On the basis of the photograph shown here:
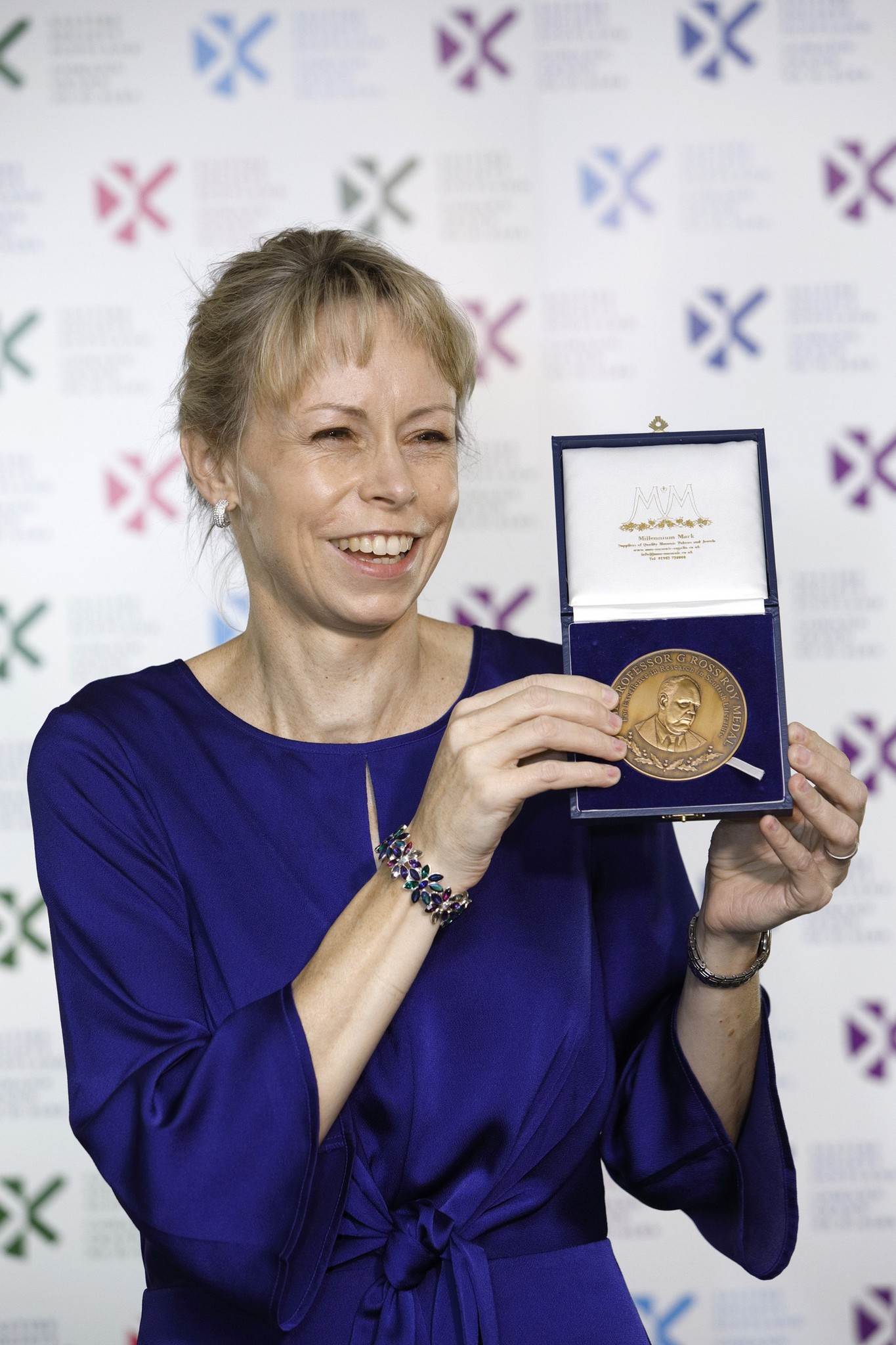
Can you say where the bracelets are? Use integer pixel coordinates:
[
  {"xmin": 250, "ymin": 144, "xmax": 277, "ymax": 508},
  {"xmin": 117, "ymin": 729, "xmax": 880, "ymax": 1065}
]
[
  {"xmin": 374, "ymin": 825, "xmax": 472, "ymax": 928},
  {"xmin": 688, "ymin": 912, "xmax": 771, "ymax": 988}
]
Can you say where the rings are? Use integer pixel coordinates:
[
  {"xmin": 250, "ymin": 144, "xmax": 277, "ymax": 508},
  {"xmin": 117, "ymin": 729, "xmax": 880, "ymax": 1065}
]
[{"xmin": 825, "ymin": 841, "xmax": 859, "ymax": 861}]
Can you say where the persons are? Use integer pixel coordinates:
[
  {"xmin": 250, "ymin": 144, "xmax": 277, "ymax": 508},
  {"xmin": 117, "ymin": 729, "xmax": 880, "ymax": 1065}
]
[{"xmin": 26, "ymin": 227, "xmax": 869, "ymax": 1345}]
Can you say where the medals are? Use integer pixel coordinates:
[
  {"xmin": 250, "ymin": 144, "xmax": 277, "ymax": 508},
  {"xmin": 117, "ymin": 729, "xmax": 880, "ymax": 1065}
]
[{"xmin": 609, "ymin": 649, "xmax": 747, "ymax": 780}]
